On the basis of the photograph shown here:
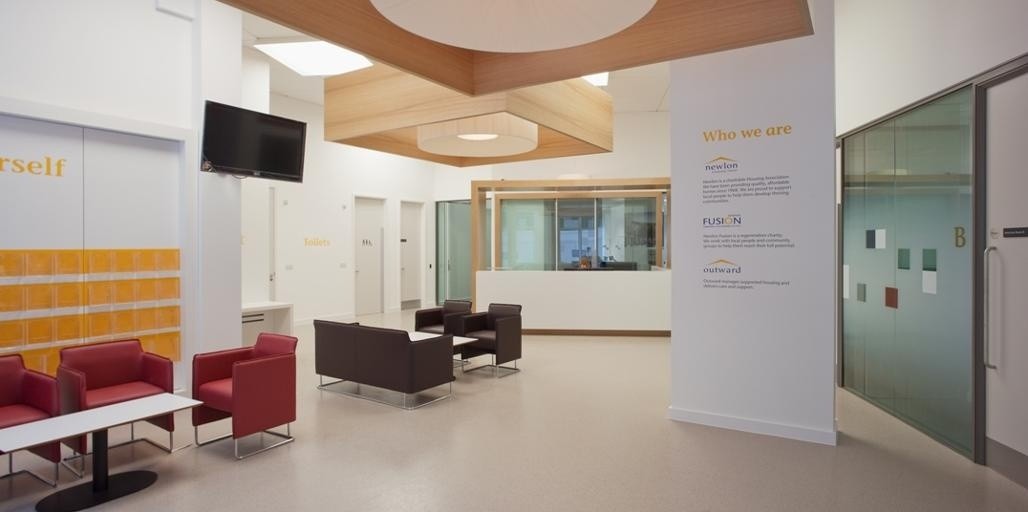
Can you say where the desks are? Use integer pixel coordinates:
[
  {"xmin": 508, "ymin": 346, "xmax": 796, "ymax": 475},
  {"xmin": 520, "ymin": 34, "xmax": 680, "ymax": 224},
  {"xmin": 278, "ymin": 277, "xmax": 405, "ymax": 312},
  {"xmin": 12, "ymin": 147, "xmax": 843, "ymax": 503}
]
[
  {"xmin": 0, "ymin": 391, "xmax": 205, "ymax": 512},
  {"xmin": 407, "ymin": 331, "xmax": 480, "ymax": 347}
]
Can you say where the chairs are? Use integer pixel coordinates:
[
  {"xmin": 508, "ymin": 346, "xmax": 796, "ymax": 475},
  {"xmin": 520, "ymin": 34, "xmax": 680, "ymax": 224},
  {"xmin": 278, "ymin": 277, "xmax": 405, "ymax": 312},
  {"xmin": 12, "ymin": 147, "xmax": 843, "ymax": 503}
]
[
  {"xmin": 192, "ymin": 331, "xmax": 299, "ymax": 460},
  {"xmin": 56, "ymin": 338, "xmax": 175, "ymax": 479},
  {"xmin": 414, "ymin": 299, "xmax": 473, "ymax": 369},
  {"xmin": 0, "ymin": 354, "xmax": 63, "ymax": 489},
  {"xmin": 460, "ymin": 303, "xmax": 522, "ymax": 378}
]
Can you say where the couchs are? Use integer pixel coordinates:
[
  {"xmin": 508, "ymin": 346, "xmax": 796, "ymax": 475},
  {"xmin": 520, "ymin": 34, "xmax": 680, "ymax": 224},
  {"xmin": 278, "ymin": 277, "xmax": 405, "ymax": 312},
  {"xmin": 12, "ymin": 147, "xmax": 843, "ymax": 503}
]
[{"xmin": 313, "ymin": 318, "xmax": 456, "ymax": 411}]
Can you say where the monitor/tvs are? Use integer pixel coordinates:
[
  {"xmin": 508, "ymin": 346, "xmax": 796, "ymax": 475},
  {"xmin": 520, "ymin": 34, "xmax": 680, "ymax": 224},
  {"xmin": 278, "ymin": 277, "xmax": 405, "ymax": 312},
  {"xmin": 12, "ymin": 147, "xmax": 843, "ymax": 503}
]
[{"xmin": 200, "ymin": 100, "xmax": 306, "ymax": 183}]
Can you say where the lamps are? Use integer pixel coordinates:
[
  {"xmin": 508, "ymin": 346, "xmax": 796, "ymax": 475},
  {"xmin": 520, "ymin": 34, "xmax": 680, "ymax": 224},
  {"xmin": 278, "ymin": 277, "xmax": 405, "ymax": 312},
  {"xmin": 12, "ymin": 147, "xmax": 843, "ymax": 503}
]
[
  {"xmin": 416, "ymin": 112, "xmax": 539, "ymax": 158},
  {"xmin": 251, "ymin": 34, "xmax": 375, "ymax": 78}
]
